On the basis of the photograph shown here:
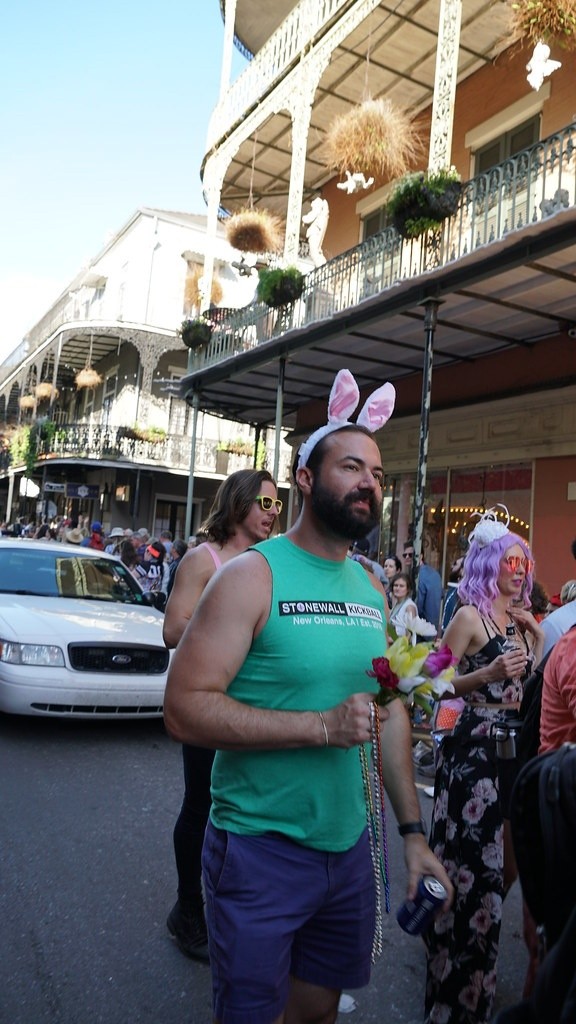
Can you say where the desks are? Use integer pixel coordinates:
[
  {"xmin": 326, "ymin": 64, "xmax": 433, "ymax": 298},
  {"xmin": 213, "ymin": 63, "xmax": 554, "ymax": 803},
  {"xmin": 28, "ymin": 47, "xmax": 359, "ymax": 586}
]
[{"xmin": 203, "ymin": 308, "xmax": 256, "ymax": 349}]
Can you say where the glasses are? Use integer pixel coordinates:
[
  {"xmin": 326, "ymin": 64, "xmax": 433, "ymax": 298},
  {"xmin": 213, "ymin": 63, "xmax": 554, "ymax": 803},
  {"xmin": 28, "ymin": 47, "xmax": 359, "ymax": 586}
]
[
  {"xmin": 501, "ymin": 556, "xmax": 534, "ymax": 576},
  {"xmin": 403, "ymin": 553, "xmax": 413, "ymax": 558},
  {"xmin": 255, "ymin": 496, "xmax": 283, "ymax": 515}
]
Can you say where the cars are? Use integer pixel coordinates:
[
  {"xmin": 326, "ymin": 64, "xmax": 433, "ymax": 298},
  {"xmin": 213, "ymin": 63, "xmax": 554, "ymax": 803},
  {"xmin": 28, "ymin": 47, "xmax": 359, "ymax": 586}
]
[{"xmin": 0, "ymin": 539, "xmax": 177, "ymax": 718}]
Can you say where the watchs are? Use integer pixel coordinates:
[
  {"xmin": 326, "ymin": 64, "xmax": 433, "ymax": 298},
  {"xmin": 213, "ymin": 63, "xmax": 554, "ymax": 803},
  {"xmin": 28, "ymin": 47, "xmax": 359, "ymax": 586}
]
[{"xmin": 399, "ymin": 818, "xmax": 426, "ymax": 836}]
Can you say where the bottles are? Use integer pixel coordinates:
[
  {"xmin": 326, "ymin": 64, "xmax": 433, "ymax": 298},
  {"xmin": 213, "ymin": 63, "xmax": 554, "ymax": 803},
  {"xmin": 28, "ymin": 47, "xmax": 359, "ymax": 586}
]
[
  {"xmin": 501, "ymin": 623, "xmax": 528, "ymax": 676},
  {"xmin": 490, "ymin": 715, "xmax": 526, "ymax": 758}
]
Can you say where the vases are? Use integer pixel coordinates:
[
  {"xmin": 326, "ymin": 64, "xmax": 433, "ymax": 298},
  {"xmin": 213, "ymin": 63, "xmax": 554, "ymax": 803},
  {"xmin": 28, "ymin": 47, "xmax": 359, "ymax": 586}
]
[
  {"xmin": 183, "ymin": 325, "xmax": 211, "ymax": 348},
  {"xmin": 392, "ymin": 181, "xmax": 459, "ymax": 240}
]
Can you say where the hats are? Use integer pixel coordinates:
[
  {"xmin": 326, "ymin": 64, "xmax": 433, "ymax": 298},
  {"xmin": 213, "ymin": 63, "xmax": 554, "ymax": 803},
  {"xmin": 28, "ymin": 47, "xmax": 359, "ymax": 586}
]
[
  {"xmin": 66, "ymin": 528, "xmax": 83, "ymax": 543},
  {"xmin": 92, "ymin": 522, "xmax": 101, "ymax": 529},
  {"xmin": 352, "ymin": 538, "xmax": 370, "ymax": 553},
  {"xmin": 108, "ymin": 528, "xmax": 124, "ymax": 537}
]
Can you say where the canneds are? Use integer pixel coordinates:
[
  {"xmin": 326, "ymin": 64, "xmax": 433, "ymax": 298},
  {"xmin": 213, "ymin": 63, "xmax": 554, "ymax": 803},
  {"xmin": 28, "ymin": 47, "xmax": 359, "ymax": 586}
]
[{"xmin": 395, "ymin": 876, "xmax": 448, "ymax": 936}]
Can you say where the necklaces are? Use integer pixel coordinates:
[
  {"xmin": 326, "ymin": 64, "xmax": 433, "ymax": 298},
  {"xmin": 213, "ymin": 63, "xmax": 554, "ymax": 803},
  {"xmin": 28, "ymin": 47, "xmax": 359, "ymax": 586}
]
[
  {"xmin": 390, "ymin": 599, "xmax": 406, "ymax": 619},
  {"xmin": 488, "ymin": 612, "xmax": 513, "ymax": 635},
  {"xmin": 358, "ymin": 701, "xmax": 390, "ymax": 963}
]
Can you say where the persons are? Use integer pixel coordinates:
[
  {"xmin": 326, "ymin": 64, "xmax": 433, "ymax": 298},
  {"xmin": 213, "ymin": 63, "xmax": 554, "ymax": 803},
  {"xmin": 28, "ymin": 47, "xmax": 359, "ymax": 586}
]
[
  {"xmin": 423, "ymin": 520, "xmax": 545, "ymax": 1024},
  {"xmin": 0, "ymin": 516, "xmax": 208, "ymax": 603},
  {"xmin": 0, "ymin": 434, "xmax": 10, "ymax": 451},
  {"xmin": 250, "ymin": 258, "xmax": 273, "ymax": 344},
  {"xmin": 538, "ymin": 538, "xmax": 576, "ymax": 659},
  {"xmin": 164, "ymin": 429, "xmax": 453, "ymax": 1024},
  {"xmin": 529, "ymin": 580, "xmax": 576, "ymax": 622},
  {"xmin": 163, "ymin": 469, "xmax": 281, "ymax": 964},
  {"xmin": 350, "ymin": 538, "xmax": 464, "ymax": 777},
  {"xmin": 519, "ymin": 625, "xmax": 576, "ymax": 999},
  {"xmin": 302, "ymin": 198, "xmax": 330, "ymax": 283}
]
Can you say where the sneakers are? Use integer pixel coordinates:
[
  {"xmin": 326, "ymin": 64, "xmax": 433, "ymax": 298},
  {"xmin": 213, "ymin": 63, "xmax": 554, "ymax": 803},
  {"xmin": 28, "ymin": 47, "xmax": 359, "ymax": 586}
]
[{"xmin": 166, "ymin": 901, "xmax": 210, "ymax": 963}]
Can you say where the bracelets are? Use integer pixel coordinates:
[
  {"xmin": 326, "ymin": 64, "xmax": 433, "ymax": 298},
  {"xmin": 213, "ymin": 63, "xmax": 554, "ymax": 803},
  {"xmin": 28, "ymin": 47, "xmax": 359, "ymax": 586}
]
[{"xmin": 318, "ymin": 711, "xmax": 328, "ymax": 747}]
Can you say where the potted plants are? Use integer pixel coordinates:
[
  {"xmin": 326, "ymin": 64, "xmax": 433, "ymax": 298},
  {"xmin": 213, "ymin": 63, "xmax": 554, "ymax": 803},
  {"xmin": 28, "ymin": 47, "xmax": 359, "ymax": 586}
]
[
  {"xmin": 214, "ymin": 439, "xmax": 254, "ymax": 475},
  {"xmin": 257, "ymin": 266, "xmax": 304, "ymax": 308}
]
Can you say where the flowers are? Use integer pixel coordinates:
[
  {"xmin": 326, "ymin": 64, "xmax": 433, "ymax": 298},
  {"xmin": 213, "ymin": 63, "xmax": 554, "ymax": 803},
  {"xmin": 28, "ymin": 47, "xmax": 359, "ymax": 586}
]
[
  {"xmin": 385, "ymin": 164, "xmax": 462, "ymax": 257},
  {"xmin": 368, "ymin": 610, "xmax": 459, "ymax": 718},
  {"xmin": 180, "ymin": 319, "xmax": 209, "ymax": 338}
]
